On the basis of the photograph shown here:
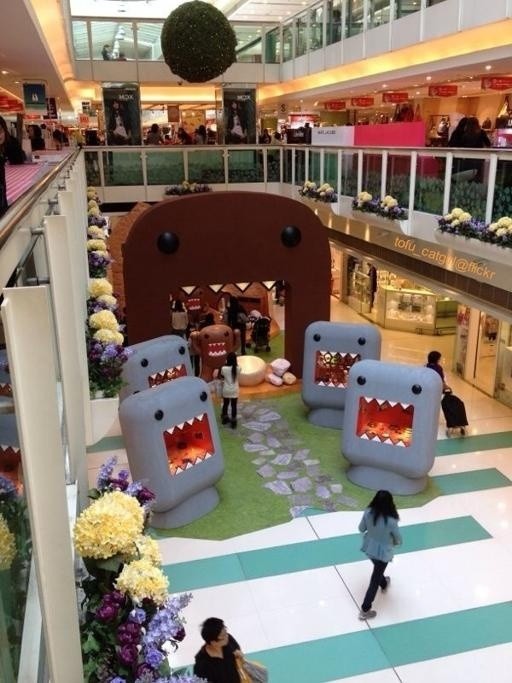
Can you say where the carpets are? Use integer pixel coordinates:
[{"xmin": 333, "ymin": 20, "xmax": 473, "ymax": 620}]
[{"xmin": 144, "ymin": 389, "xmax": 445, "ymax": 542}]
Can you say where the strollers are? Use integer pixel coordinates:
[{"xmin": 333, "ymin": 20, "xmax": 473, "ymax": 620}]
[{"xmin": 246, "ymin": 314, "xmax": 273, "ymax": 353}]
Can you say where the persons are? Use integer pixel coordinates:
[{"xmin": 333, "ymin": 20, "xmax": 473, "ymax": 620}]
[
  {"xmin": 226, "ymin": 296, "xmax": 272, "ymax": 355},
  {"xmin": 423, "ymin": 350, "xmax": 445, "ymax": 386},
  {"xmin": 430, "ymin": 112, "xmax": 512, "ymax": 183},
  {"xmin": 28, "ymin": 125, "xmax": 45, "ymax": 151},
  {"xmin": 367, "ymin": 262, "xmax": 378, "ymax": 314},
  {"xmin": 194, "ymin": 616, "xmax": 242, "ymax": 682},
  {"xmin": 172, "ymin": 297, "xmax": 216, "ymax": 376},
  {"xmin": 143, "ymin": 124, "xmax": 170, "ymax": 144},
  {"xmin": 225, "ymin": 103, "xmax": 247, "ymax": 143},
  {"xmin": 178, "ymin": 124, "xmax": 215, "ymax": 144},
  {"xmin": 107, "ymin": 103, "xmax": 132, "ymax": 145},
  {"xmin": 259, "ymin": 125, "xmax": 286, "ymax": 144},
  {"xmin": 221, "ymin": 352, "xmax": 242, "ymax": 428},
  {"xmin": 1, "ymin": 119, "xmax": 26, "ymax": 164},
  {"xmin": 355, "ymin": 489, "xmax": 402, "ymax": 619}
]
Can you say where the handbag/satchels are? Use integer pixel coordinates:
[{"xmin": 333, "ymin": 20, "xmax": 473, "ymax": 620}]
[
  {"xmin": 237, "ymin": 312, "xmax": 249, "ymax": 323},
  {"xmin": 234, "ymin": 657, "xmax": 254, "ymax": 683},
  {"xmin": 242, "ymin": 660, "xmax": 268, "ymax": 683}
]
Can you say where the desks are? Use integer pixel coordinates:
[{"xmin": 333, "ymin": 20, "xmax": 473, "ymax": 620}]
[
  {"xmin": 4, "ymin": 162, "xmax": 48, "ymax": 207},
  {"xmin": 237, "ymin": 354, "xmax": 266, "ymax": 387}
]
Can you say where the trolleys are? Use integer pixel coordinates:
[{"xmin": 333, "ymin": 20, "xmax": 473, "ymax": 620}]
[{"xmin": 441, "ymin": 387, "xmax": 468, "ymax": 437}]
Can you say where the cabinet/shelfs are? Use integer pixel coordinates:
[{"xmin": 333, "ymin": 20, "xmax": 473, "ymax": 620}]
[
  {"xmin": 348, "ymin": 267, "xmax": 372, "ymax": 314},
  {"xmin": 375, "ymin": 269, "xmax": 415, "ymax": 290},
  {"xmin": 377, "ymin": 284, "xmax": 436, "ymax": 335}
]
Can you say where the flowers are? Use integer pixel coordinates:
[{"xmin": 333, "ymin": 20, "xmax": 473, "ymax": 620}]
[
  {"xmin": 434, "ymin": 206, "xmax": 512, "ymax": 247},
  {"xmin": 87, "ymin": 185, "xmax": 132, "ymax": 399},
  {"xmin": 165, "ymin": 181, "xmax": 210, "ymax": 195},
  {"xmin": 68, "ymin": 455, "xmax": 207, "ymax": 682},
  {"xmin": 0, "ymin": 474, "xmax": 32, "ymax": 683},
  {"xmin": 297, "ymin": 180, "xmax": 338, "ymax": 203},
  {"xmin": 349, "ymin": 191, "xmax": 408, "ymax": 221}
]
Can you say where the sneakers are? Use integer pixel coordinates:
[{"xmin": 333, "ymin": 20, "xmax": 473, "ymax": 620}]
[
  {"xmin": 221, "ymin": 417, "xmax": 231, "ymax": 424},
  {"xmin": 358, "ymin": 607, "xmax": 377, "ymax": 621},
  {"xmin": 231, "ymin": 420, "xmax": 237, "ymax": 428},
  {"xmin": 380, "ymin": 576, "xmax": 391, "ymax": 593}
]
